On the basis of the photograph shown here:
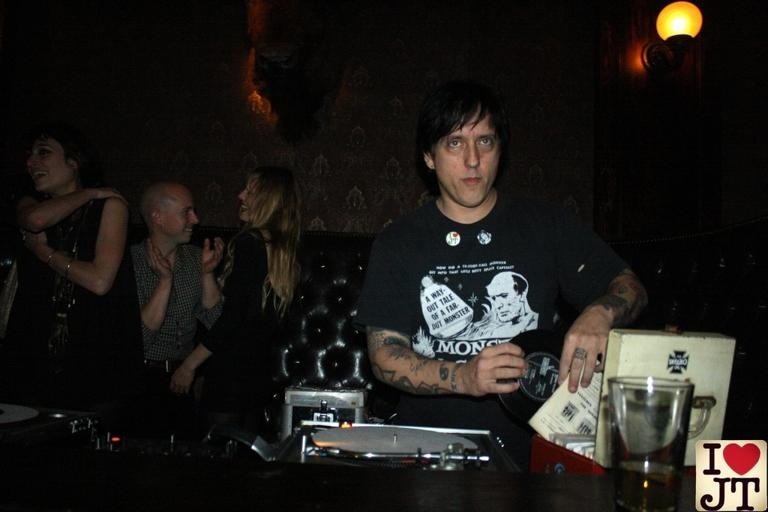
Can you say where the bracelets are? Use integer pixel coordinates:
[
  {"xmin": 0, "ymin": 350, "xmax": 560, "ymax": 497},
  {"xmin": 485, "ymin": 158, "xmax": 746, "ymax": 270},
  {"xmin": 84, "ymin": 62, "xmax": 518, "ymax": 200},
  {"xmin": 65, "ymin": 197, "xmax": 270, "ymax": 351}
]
[
  {"xmin": 64, "ymin": 257, "xmax": 74, "ymax": 281},
  {"xmin": 47, "ymin": 249, "xmax": 57, "ymax": 267}
]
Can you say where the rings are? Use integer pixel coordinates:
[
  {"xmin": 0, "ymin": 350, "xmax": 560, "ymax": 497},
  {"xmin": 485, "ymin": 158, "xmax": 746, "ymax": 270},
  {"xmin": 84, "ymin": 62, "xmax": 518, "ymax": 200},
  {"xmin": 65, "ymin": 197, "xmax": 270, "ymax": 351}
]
[{"xmin": 574, "ymin": 346, "xmax": 586, "ymax": 360}]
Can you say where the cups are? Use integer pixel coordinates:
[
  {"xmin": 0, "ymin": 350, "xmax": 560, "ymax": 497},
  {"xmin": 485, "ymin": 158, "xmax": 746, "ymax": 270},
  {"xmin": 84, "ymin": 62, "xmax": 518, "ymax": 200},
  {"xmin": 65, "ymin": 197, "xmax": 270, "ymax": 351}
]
[{"xmin": 609, "ymin": 376, "xmax": 710, "ymax": 512}]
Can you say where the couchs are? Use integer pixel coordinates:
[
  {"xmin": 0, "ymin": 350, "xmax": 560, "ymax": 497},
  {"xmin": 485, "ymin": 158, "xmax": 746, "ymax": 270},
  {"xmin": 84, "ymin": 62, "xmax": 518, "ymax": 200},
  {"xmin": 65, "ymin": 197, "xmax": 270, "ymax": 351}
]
[{"xmin": 128, "ymin": 214, "xmax": 768, "ymax": 447}]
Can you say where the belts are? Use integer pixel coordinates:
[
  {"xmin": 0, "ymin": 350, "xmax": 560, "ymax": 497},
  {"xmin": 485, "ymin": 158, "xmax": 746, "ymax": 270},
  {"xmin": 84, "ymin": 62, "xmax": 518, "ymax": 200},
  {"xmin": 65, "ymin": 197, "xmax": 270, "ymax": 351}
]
[{"xmin": 144, "ymin": 359, "xmax": 184, "ymax": 373}]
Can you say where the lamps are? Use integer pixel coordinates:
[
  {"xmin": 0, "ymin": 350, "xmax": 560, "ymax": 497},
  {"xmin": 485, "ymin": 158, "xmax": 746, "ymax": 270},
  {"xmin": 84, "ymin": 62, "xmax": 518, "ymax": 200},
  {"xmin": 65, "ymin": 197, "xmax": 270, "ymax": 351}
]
[{"xmin": 642, "ymin": 0, "xmax": 703, "ymax": 77}]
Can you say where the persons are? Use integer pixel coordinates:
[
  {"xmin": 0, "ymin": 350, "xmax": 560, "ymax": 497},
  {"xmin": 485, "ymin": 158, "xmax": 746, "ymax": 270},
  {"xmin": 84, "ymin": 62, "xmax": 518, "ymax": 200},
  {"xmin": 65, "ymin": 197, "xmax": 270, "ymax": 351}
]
[
  {"xmin": 130, "ymin": 181, "xmax": 225, "ymax": 437},
  {"xmin": 170, "ymin": 167, "xmax": 305, "ymax": 428},
  {"xmin": 13, "ymin": 120, "xmax": 133, "ymax": 443},
  {"xmin": 356, "ymin": 78, "xmax": 650, "ymax": 479}
]
